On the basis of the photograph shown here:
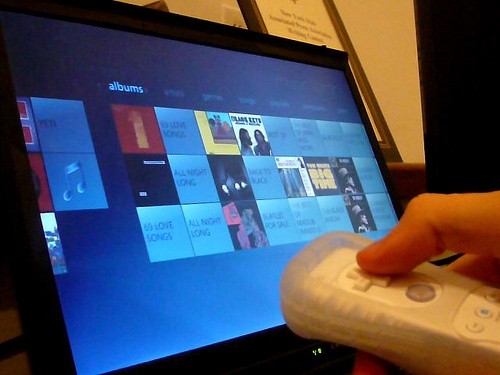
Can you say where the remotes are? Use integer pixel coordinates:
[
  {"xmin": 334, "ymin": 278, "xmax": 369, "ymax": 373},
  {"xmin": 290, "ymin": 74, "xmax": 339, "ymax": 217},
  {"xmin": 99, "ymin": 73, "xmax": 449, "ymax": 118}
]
[{"xmin": 280, "ymin": 230, "xmax": 499, "ymax": 370}]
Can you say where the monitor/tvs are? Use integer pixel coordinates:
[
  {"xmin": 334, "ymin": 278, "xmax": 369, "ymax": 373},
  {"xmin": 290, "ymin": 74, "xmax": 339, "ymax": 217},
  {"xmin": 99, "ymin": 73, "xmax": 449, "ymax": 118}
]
[{"xmin": 1, "ymin": 1, "xmax": 406, "ymax": 375}]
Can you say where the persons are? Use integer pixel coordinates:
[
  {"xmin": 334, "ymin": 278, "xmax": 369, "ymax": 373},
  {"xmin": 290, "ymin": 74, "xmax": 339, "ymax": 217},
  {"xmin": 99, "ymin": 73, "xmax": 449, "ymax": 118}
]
[
  {"xmin": 339, "ymin": 168, "xmax": 358, "ymax": 194},
  {"xmin": 208, "ymin": 118, "xmax": 218, "ymax": 140},
  {"xmin": 352, "ymin": 190, "xmax": 500, "ymax": 375},
  {"xmin": 238, "ymin": 128, "xmax": 254, "ymax": 156},
  {"xmin": 237, "ymin": 208, "xmax": 269, "ymax": 249},
  {"xmin": 254, "ymin": 130, "xmax": 271, "ymax": 156},
  {"xmin": 352, "ymin": 204, "xmax": 372, "ymax": 232},
  {"xmin": 218, "ymin": 121, "xmax": 235, "ymax": 140}
]
